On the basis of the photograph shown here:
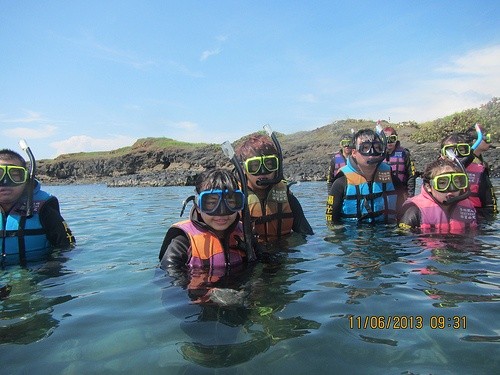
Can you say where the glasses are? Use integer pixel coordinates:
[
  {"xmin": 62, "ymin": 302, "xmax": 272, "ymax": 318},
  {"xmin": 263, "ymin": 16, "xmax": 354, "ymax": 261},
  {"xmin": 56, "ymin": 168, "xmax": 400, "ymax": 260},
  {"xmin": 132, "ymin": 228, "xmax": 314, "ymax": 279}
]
[
  {"xmin": 485, "ymin": 133, "xmax": 492, "ymax": 143},
  {"xmin": 340, "ymin": 140, "xmax": 353, "ymax": 147},
  {"xmin": 433, "ymin": 172, "xmax": 469, "ymax": 192},
  {"xmin": 0, "ymin": 165, "xmax": 29, "ymax": 184},
  {"xmin": 245, "ymin": 154, "xmax": 279, "ymax": 174},
  {"xmin": 199, "ymin": 188, "xmax": 245, "ymax": 214},
  {"xmin": 441, "ymin": 143, "xmax": 471, "ymax": 159},
  {"xmin": 382, "ymin": 134, "xmax": 397, "ymax": 144},
  {"xmin": 359, "ymin": 141, "xmax": 385, "ymax": 156}
]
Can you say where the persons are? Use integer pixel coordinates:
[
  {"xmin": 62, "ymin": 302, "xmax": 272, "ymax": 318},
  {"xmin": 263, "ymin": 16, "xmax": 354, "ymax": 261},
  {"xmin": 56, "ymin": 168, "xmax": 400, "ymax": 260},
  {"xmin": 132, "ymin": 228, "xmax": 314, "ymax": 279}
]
[
  {"xmin": 158, "ymin": 141, "xmax": 257, "ymax": 274},
  {"xmin": 325, "ymin": 120, "xmax": 418, "ymax": 229},
  {"xmin": 0, "ymin": 140, "xmax": 76, "ymax": 255},
  {"xmin": 437, "ymin": 123, "xmax": 498, "ymax": 217},
  {"xmin": 397, "ymin": 155, "xmax": 479, "ymax": 229},
  {"xmin": 233, "ymin": 123, "xmax": 314, "ymax": 246}
]
